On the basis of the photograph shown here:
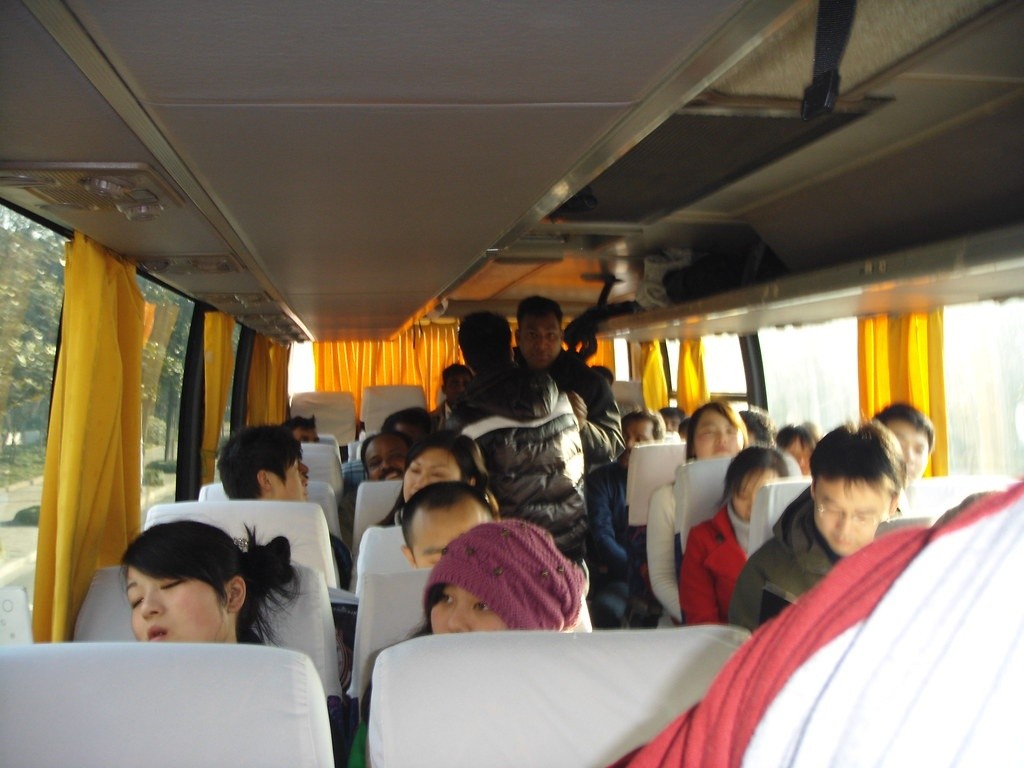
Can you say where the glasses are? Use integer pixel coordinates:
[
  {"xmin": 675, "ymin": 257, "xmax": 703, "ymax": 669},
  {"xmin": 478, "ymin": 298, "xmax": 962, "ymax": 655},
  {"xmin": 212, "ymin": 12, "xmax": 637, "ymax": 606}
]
[{"xmin": 812, "ymin": 491, "xmax": 880, "ymax": 528}]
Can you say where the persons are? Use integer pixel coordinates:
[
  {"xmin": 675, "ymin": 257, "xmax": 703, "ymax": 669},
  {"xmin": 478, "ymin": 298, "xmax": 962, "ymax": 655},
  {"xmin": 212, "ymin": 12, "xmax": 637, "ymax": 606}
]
[
  {"xmin": 428, "ymin": 363, "xmax": 474, "ymax": 433},
  {"xmin": 118, "ymin": 520, "xmax": 301, "ymax": 644},
  {"xmin": 511, "ymin": 294, "xmax": 647, "ymax": 629},
  {"xmin": 347, "ymin": 518, "xmax": 585, "ymax": 768},
  {"xmin": 381, "ymin": 406, "xmax": 432, "ymax": 445},
  {"xmin": 585, "ymin": 412, "xmax": 666, "ymax": 628},
  {"xmin": 216, "ymin": 423, "xmax": 353, "ymax": 592},
  {"xmin": 348, "ymin": 429, "xmax": 500, "ymax": 595},
  {"xmin": 339, "ymin": 430, "xmax": 412, "ymax": 551},
  {"xmin": 399, "ymin": 479, "xmax": 495, "ymax": 570},
  {"xmin": 277, "ymin": 413, "xmax": 319, "ymax": 444},
  {"xmin": 591, "ymin": 366, "xmax": 614, "ymax": 387},
  {"xmin": 440, "ymin": 310, "xmax": 628, "ymax": 566},
  {"xmin": 657, "ymin": 400, "xmax": 935, "ymax": 634}
]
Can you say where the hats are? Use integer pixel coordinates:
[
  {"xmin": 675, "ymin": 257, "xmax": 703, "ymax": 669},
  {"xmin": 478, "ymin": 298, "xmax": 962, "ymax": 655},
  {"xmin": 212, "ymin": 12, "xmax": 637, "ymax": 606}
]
[{"xmin": 424, "ymin": 518, "xmax": 586, "ymax": 632}]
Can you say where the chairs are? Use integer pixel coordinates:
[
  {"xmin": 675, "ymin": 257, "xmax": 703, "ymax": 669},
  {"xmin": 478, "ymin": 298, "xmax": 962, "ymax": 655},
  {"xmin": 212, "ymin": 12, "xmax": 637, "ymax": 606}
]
[{"xmin": 0, "ymin": 383, "xmax": 976, "ymax": 768}]
